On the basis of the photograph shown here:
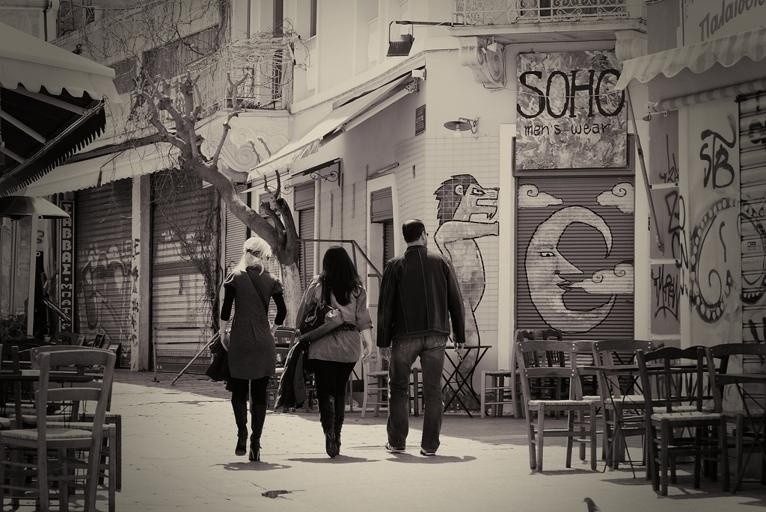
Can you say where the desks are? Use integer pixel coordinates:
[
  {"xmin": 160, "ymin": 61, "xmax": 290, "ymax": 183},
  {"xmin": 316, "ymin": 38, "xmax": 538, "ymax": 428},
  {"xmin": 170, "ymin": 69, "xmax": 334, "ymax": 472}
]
[{"xmin": 420, "ymin": 344, "xmax": 493, "ymax": 418}]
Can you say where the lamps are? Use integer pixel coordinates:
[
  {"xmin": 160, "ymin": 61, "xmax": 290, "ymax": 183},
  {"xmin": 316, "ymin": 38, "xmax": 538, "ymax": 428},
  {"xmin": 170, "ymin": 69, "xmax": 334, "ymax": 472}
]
[{"xmin": 443, "ymin": 116, "xmax": 478, "ymax": 139}]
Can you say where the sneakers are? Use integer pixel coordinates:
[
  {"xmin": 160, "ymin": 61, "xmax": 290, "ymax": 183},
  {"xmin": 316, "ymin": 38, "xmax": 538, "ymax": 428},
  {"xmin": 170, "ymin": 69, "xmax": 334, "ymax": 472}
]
[
  {"xmin": 384, "ymin": 442, "xmax": 405, "ymax": 453},
  {"xmin": 420, "ymin": 446, "xmax": 436, "ymax": 456}
]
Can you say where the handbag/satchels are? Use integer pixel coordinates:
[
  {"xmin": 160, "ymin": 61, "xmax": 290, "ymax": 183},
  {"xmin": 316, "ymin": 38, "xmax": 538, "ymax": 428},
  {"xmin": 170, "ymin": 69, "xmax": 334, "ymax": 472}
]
[{"xmin": 299, "ymin": 275, "xmax": 344, "ymax": 342}]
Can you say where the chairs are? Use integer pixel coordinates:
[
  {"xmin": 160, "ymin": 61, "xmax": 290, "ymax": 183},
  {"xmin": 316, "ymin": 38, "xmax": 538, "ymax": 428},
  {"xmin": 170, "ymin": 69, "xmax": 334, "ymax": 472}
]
[
  {"xmin": 264, "ymin": 328, "xmax": 321, "ymax": 411},
  {"xmin": 360, "ymin": 327, "xmax": 424, "ymax": 417},
  {"xmin": 1, "ymin": 334, "xmax": 122, "ymax": 511}
]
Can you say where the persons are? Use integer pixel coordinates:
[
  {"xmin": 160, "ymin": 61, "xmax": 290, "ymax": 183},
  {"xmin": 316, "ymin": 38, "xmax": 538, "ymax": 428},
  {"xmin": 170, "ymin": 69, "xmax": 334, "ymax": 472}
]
[
  {"xmin": 293, "ymin": 243, "xmax": 374, "ymax": 460},
  {"xmin": 218, "ymin": 235, "xmax": 287, "ymax": 465},
  {"xmin": 376, "ymin": 218, "xmax": 466, "ymax": 458}
]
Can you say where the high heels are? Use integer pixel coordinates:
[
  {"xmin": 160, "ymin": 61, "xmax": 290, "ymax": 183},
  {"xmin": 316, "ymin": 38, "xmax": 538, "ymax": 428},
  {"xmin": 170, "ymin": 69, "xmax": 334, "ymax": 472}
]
[
  {"xmin": 325, "ymin": 427, "xmax": 338, "ymax": 458},
  {"xmin": 235, "ymin": 431, "xmax": 247, "ymax": 456},
  {"xmin": 248, "ymin": 442, "xmax": 260, "ymax": 462}
]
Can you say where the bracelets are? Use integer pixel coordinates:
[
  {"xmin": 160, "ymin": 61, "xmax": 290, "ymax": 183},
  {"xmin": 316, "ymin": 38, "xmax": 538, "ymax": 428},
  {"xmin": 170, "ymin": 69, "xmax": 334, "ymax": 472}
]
[{"xmin": 218, "ymin": 328, "xmax": 227, "ymax": 335}]
